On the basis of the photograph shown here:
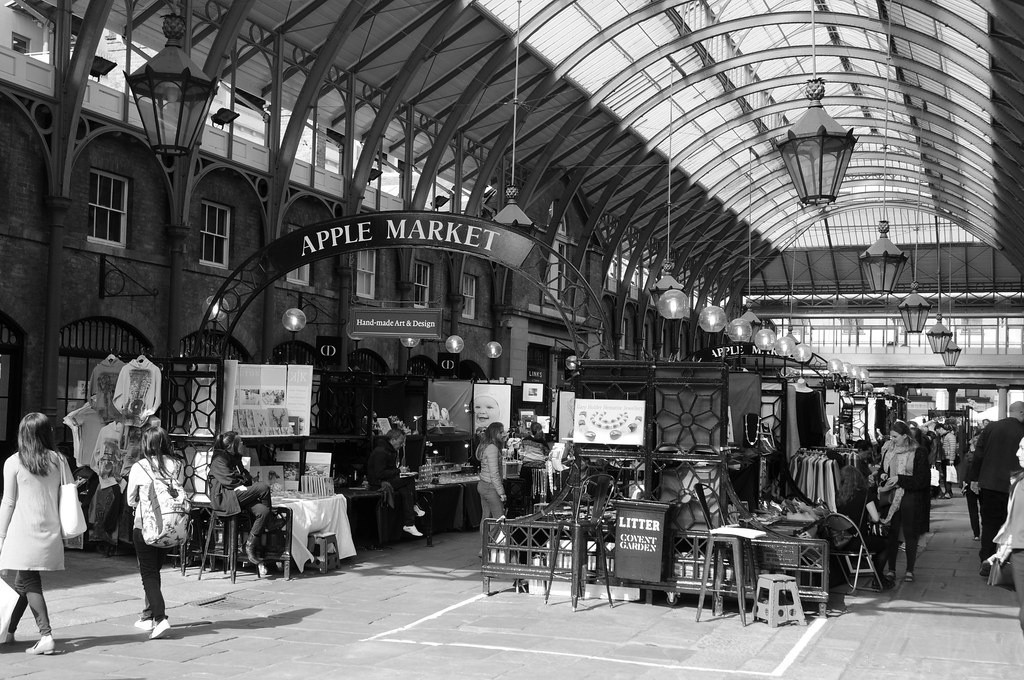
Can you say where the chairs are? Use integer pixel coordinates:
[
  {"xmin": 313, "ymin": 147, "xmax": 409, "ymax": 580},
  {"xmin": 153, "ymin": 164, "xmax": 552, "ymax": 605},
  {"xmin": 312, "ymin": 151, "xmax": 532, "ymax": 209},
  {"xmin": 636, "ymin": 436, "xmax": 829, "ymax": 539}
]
[
  {"xmin": 691, "ymin": 482, "xmax": 758, "ymax": 624},
  {"xmin": 821, "ymin": 512, "xmax": 881, "ymax": 596},
  {"xmin": 542, "ymin": 474, "xmax": 614, "ymax": 612}
]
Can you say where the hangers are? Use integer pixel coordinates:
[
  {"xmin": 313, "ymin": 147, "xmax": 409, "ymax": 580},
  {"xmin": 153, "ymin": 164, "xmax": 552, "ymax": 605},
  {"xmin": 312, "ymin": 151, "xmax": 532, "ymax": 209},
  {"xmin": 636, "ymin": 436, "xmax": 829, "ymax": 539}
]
[
  {"xmin": 135, "ymin": 347, "xmax": 146, "ymax": 363},
  {"xmin": 106, "ymin": 346, "xmax": 114, "ymax": 362},
  {"xmin": 796, "ymin": 448, "xmax": 858, "ymax": 458}
]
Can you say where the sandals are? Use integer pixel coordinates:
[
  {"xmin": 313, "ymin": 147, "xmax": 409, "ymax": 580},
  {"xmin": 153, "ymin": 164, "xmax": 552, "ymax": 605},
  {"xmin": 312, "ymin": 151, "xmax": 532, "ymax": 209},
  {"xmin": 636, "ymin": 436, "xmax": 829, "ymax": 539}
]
[
  {"xmin": 903, "ymin": 571, "xmax": 915, "ymax": 583},
  {"xmin": 885, "ymin": 570, "xmax": 896, "ymax": 581}
]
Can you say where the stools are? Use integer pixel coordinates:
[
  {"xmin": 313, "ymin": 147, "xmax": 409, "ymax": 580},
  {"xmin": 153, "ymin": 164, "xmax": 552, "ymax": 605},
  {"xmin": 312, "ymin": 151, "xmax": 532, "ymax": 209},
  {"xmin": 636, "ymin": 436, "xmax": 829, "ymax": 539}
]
[
  {"xmin": 195, "ymin": 509, "xmax": 260, "ymax": 585},
  {"xmin": 303, "ymin": 532, "xmax": 341, "ymax": 574},
  {"xmin": 750, "ymin": 572, "xmax": 808, "ymax": 628}
]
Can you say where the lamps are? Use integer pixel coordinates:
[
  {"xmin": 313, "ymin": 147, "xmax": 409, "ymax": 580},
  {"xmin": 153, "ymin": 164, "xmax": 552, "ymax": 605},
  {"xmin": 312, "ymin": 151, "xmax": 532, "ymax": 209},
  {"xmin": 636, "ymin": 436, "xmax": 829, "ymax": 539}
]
[
  {"xmin": 366, "ymin": 168, "xmax": 383, "ymax": 186},
  {"xmin": 89, "ymin": 55, "xmax": 118, "ymax": 82},
  {"xmin": 429, "ymin": 196, "xmax": 449, "ymax": 212},
  {"xmin": 210, "ymin": 108, "xmax": 239, "ymax": 130},
  {"xmin": 655, "ymin": 2, "xmax": 963, "ymax": 384}
]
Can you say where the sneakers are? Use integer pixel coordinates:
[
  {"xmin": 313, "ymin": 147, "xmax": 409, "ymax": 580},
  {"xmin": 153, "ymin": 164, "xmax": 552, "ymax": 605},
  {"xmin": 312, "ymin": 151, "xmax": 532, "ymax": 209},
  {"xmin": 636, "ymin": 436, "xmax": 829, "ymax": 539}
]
[
  {"xmin": 134, "ymin": 619, "xmax": 153, "ymax": 631},
  {"xmin": 403, "ymin": 525, "xmax": 423, "ymax": 536},
  {"xmin": 414, "ymin": 505, "xmax": 425, "ymax": 517},
  {"xmin": 149, "ymin": 618, "xmax": 171, "ymax": 639}
]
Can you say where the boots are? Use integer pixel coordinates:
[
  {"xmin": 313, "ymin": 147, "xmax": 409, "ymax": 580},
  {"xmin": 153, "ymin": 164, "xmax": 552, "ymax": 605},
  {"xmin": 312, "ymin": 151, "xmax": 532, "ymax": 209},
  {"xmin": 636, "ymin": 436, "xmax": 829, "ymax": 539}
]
[
  {"xmin": 263, "ymin": 507, "xmax": 287, "ymax": 532},
  {"xmin": 238, "ymin": 531, "xmax": 263, "ymax": 565}
]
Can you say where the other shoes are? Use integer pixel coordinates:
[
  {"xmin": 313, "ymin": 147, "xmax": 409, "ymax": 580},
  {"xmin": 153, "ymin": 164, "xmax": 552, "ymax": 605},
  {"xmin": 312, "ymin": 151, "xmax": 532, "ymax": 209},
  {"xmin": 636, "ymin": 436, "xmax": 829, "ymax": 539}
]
[
  {"xmin": 944, "ymin": 493, "xmax": 951, "ymax": 499},
  {"xmin": 979, "ymin": 560, "xmax": 991, "ymax": 577},
  {"xmin": 478, "ymin": 549, "xmax": 483, "ymax": 558},
  {"xmin": 973, "ymin": 536, "xmax": 980, "ymax": 542},
  {"xmin": 25, "ymin": 640, "xmax": 55, "ymax": 655},
  {"xmin": 6, "ymin": 631, "xmax": 16, "ymax": 643}
]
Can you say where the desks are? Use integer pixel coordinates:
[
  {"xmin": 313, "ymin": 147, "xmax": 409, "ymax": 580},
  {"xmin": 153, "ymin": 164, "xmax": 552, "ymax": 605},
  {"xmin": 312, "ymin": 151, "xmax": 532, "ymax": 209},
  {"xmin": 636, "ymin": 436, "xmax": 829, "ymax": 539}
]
[{"xmin": 335, "ymin": 474, "xmax": 523, "ymax": 545}]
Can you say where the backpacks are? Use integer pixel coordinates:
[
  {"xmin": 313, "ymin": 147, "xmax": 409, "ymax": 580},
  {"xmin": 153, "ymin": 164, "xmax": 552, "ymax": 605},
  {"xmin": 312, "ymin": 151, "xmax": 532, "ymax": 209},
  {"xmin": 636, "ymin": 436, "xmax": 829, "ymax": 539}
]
[{"xmin": 136, "ymin": 460, "xmax": 190, "ymax": 548}]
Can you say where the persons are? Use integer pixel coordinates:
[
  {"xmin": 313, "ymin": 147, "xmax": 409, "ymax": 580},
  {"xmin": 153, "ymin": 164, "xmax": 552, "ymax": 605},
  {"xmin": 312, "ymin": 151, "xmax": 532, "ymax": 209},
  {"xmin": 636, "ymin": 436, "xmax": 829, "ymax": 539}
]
[
  {"xmin": 366, "ymin": 429, "xmax": 425, "ymax": 536},
  {"xmin": 970, "ymin": 401, "xmax": 1024, "ymax": 576},
  {"xmin": 835, "ymin": 465, "xmax": 896, "ymax": 590},
  {"xmin": 474, "ymin": 396, "xmax": 500, "ymax": 427},
  {"xmin": 126, "ymin": 425, "xmax": 184, "ymax": 640},
  {"xmin": 877, "ymin": 421, "xmax": 931, "ymax": 582},
  {"xmin": 207, "ymin": 431, "xmax": 287, "ymax": 566},
  {"xmin": 959, "ymin": 434, "xmax": 981, "ymax": 541},
  {"xmin": 933, "ymin": 423, "xmax": 956, "ymax": 499},
  {"xmin": 924, "ymin": 431, "xmax": 941, "ymax": 500},
  {"xmin": 477, "ymin": 422, "xmax": 507, "ymax": 558},
  {"xmin": 519, "ymin": 422, "xmax": 551, "ymax": 503},
  {"xmin": 793, "ymin": 379, "xmax": 825, "ymax": 455},
  {"xmin": 0, "ymin": 411, "xmax": 77, "ymax": 656},
  {"xmin": 986, "ymin": 436, "xmax": 1024, "ymax": 636}
]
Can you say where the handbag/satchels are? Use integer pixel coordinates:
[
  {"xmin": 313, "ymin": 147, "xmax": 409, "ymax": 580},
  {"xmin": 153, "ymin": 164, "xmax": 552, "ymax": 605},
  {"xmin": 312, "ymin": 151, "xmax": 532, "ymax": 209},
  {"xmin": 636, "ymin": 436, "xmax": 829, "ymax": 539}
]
[
  {"xmin": 58, "ymin": 452, "xmax": 86, "ymax": 540},
  {"xmin": 946, "ymin": 463, "xmax": 958, "ymax": 483},
  {"xmin": 930, "ymin": 465, "xmax": 939, "ymax": 487},
  {"xmin": 986, "ymin": 559, "xmax": 1015, "ymax": 594}
]
